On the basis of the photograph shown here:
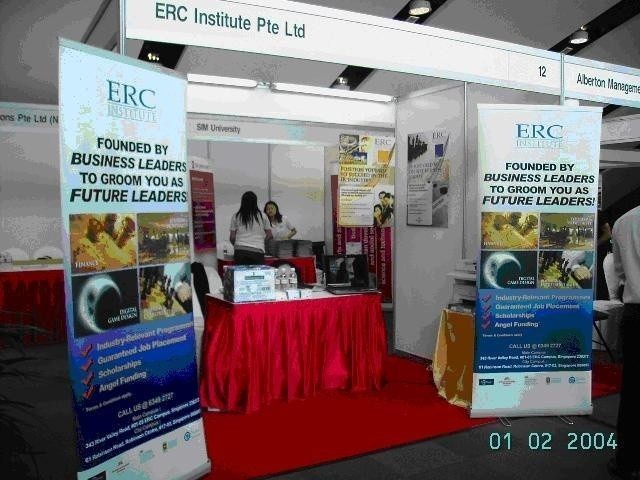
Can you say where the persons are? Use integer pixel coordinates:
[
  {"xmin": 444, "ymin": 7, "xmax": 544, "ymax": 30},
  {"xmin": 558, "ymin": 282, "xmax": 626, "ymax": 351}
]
[
  {"xmin": 374, "ymin": 191, "xmax": 395, "ymax": 228},
  {"xmin": 607, "ymin": 205, "xmax": 640, "ymax": 480},
  {"xmin": 264, "ymin": 201, "xmax": 297, "ymax": 240},
  {"xmin": 228, "ymin": 190, "xmax": 273, "ymax": 266},
  {"xmin": 591, "ymin": 210, "xmax": 614, "ymax": 352}
]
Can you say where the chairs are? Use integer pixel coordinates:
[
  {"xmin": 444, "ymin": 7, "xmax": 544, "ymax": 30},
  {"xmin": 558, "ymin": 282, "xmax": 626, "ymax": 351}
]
[{"xmin": 191, "ymin": 262, "xmax": 211, "ymax": 319}]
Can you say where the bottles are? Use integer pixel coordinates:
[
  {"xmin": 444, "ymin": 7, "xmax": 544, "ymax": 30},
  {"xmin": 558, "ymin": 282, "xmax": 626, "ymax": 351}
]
[
  {"xmin": 274, "ymin": 268, "xmax": 281, "ymax": 289},
  {"xmin": 288, "ymin": 267, "xmax": 297, "ymax": 290},
  {"xmin": 280, "ymin": 267, "xmax": 287, "ymax": 290}
]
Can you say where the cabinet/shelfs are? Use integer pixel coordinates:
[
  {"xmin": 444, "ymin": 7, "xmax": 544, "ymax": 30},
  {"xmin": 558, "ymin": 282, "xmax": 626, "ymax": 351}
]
[{"xmin": 432, "ymin": 271, "xmax": 476, "ymax": 410}]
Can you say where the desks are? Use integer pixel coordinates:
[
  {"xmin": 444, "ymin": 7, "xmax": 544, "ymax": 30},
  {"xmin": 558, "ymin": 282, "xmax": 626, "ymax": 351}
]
[
  {"xmin": 199, "ymin": 288, "xmax": 389, "ymax": 415},
  {"xmin": 0, "ymin": 264, "xmax": 67, "ymax": 347},
  {"xmin": 217, "ymin": 256, "xmax": 317, "ymax": 284}
]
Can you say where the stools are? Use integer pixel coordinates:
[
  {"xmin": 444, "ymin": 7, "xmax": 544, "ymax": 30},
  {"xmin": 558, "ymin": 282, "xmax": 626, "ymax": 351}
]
[{"xmin": 592, "ymin": 310, "xmax": 616, "ymax": 363}]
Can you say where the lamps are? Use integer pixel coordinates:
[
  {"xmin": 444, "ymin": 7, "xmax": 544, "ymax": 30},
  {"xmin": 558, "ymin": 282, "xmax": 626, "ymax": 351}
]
[
  {"xmin": 408, "ymin": 0, "xmax": 432, "ymax": 16},
  {"xmin": 569, "ymin": 31, "xmax": 588, "ymax": 45}
]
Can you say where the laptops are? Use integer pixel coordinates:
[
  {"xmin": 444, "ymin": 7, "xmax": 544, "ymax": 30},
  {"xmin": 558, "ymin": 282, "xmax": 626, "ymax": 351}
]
[{"xmin": 324, "ymin": 254, "xmax": 378, "ymax": 294}]
